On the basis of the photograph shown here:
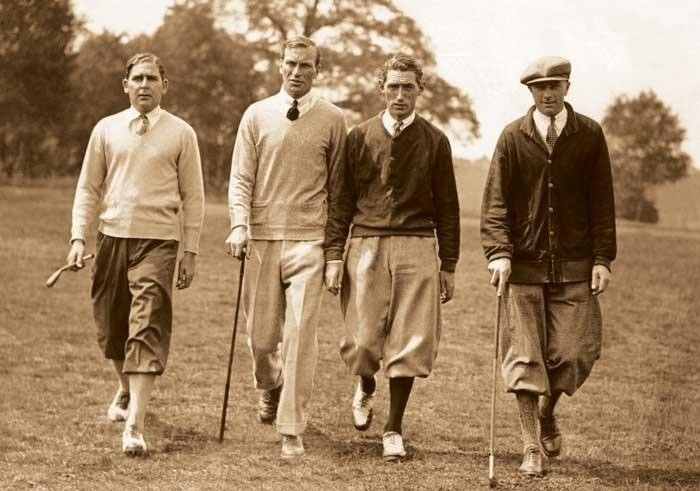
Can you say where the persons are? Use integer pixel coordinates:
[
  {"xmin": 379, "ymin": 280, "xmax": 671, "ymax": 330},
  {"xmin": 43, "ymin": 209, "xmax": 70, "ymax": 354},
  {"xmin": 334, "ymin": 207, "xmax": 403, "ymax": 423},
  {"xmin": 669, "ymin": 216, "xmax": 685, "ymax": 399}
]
[
  {"xmin": 320, "ymin": 57, "xmax": 460, "ymax": 463},
  {"xmin": 480, "ymin": 56, "xmax": 617, "ymax": 476},
  {"xmin": 224, "ymin": 36, "xmax": 347, "ymax": 462},
  {"xmin": 67, "ymin": 52, "xmax": 203, "ymax": 454}
]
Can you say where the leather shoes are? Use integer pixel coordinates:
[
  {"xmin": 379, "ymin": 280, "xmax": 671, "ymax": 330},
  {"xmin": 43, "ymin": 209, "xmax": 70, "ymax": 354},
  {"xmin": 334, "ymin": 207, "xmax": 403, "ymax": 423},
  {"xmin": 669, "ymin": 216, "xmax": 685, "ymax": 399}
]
[
  {"xmin": 519, "ymin": 447, "xmax": 543, "ymax": 477},
  {"xmin": 259, "ymin": 378, "xmax": 283, "ymax": 424},
  {"xmin": 121, "ymin": 426, "xmax": 146, "ymax": 454},
  {"xmin": 281, "ymin": 434, "xmax": 303, "ymax": 459},
  {"xmin": 539, "ymin": 413, "xmax": 562, "ymax": 457},
  {"xmin": 351, "ymin": 382, "xmax": 375, "ymax": 430},
  {"xmin": 108, "ymin": 391, "xmax": 128, "ymax": 422},
  {"xmin": 381, "ymin": 431, "xmax": 406, "ymax": 462}
]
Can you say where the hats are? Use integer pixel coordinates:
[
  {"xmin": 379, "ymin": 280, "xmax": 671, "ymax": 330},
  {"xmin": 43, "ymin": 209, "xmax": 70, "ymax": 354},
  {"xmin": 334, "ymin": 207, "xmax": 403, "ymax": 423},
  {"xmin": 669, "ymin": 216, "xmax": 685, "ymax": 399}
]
[{"xmin": 519, "ymin": 57, "xmax": 572, "ymax": 85}]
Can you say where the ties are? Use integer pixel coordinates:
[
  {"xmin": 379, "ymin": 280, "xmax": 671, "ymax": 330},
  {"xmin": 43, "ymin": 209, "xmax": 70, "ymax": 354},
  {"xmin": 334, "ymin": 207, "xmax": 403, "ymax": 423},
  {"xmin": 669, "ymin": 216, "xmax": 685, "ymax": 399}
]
[
  {"xmin": 287, "ymin": 100, "xmax": 299, "ymax": 119},
  {"xmin": 546, "ymin": 116, "xmax": 559, "ymax": 150},
  {"xmin": 393, "ymin": 119, "xmax": 403, "ymax": 136},
  {"xmin": 136, "ymin": 115, "xmax": 149, "ymax": 135}
]
